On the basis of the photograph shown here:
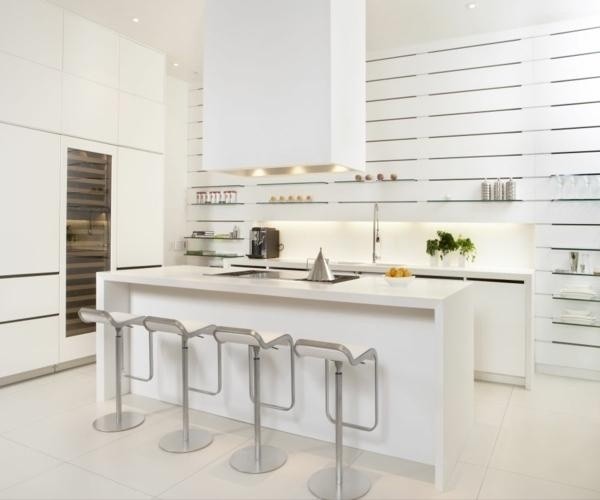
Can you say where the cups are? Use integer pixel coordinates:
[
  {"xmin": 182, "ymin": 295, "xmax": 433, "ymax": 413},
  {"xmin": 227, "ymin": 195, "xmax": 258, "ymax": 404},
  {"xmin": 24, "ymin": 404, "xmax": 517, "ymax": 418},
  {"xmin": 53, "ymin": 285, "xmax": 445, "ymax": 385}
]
[{"xmin": 569, "ymin": 251, "xmax": 579, "ymax": 271}]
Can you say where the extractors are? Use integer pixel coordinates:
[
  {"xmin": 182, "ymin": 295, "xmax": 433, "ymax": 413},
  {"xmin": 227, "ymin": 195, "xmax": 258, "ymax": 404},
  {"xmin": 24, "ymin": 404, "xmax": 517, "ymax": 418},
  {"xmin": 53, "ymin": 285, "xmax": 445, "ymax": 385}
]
[{"xmin": 203, "ymin": 0, "xmax": 367, "ymax": 178}]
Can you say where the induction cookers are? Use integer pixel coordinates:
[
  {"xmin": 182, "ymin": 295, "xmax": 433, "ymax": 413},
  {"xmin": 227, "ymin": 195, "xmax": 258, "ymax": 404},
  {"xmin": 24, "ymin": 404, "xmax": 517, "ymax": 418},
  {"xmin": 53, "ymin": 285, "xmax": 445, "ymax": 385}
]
[{"xmin": 206, "ymin": 269, "xmax": 360, "ymax": 284}]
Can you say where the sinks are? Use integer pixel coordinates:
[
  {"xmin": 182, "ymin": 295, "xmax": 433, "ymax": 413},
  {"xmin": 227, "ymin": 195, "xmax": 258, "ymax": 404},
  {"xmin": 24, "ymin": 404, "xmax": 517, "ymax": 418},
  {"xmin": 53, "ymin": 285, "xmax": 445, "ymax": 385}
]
[
  {"xmin": 239, "ymin": 270, "xmax": 311, "ymax": 280},
  {"xmin": 336, "ymin": 261, "xmax": 407, "ymax": 269}
]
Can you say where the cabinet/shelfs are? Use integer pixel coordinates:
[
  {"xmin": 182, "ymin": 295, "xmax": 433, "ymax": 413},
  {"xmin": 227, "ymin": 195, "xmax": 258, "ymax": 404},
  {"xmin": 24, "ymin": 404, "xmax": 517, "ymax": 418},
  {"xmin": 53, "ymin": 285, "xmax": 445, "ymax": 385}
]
[
  {"xmin": 545, "ymin": 25, "xmax": 600, "ymax": 348},
  {"xmin": 463, "ymin": 272, "xmax": 535, "ymax": 389},
  {"xmin": 187, "ymin": 83, "xmax": 246, "ymax": 260},
  {"xmin": 426, "ymin": 35, "xmax": 523, "ymax": 201},
  {"xmin": 335, "ymin": 53, "xmax": 420, "ymax": 205},
  {"xmin": 255, "ymin": 182, "xmax": 327, "ymax": 205}
]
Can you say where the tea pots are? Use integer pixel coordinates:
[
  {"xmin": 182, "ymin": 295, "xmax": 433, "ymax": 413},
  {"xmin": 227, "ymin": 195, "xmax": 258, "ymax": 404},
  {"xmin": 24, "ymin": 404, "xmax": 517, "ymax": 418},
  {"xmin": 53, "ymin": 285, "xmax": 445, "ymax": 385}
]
[{"xmin": 303, "ymin": 247, "xmax": 335, "ymax": 281}]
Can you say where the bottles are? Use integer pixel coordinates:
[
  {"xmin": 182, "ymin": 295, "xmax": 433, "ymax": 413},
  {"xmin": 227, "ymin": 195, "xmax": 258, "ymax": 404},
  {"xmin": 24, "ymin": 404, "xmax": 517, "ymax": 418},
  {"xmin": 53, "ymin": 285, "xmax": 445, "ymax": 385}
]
[
  {"xmin": 233, "ymin": 225, "xmax": 240, "ymax": 238},
  {"xmin": 211, "ymin": 191, "xmax": 215, "ymax": 204},
  {"xmin": 197, "ymin": 192, "xmax": 201, "ymax": 204},
  {"xmin": 224, "ymin": 192, "xmax": 231, "ymax": 203},
  {"xmin": 215, "ymin": 192, "xmax": 221, "ymax": 204},
  {"xmin": 200, "ymin": 192, "xmax": 206, "ymax": 203},
  {"xmin": 230, "ymin": 191, "xmax": 236, "ymax": 203}
]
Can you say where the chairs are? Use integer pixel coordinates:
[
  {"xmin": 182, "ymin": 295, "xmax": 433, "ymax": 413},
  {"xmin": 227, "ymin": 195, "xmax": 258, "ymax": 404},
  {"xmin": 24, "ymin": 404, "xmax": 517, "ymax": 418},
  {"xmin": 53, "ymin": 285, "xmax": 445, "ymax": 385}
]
[
  {"xmin": 142, "ymin": 316, "xmax": 224, "ymax": 454},
  {"xmin": 213, "ymin": 326, "xmax": 294, "ymax": 474},
  {"xmin": 77, "ymin": 307, "xmax": 151, "ymax": 433},
  {"xmin": 295, "ymin": 339, "xmax": 379, "ymax": 500}
]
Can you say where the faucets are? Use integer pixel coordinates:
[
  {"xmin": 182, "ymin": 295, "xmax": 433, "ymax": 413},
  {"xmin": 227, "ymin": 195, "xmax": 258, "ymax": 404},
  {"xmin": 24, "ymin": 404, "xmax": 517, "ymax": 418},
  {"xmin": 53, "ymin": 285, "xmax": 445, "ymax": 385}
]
[{"xmin": 372, "ymin": 202, "xmax": 380, "ymax": 262}]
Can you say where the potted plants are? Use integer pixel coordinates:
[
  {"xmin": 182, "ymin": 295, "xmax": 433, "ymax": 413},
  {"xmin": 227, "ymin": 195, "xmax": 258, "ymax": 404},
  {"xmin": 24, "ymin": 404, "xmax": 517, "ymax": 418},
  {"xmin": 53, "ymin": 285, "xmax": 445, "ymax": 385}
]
[{"xmin": 426, "ymin": 232, "xmax": 475, "ymax": 267}]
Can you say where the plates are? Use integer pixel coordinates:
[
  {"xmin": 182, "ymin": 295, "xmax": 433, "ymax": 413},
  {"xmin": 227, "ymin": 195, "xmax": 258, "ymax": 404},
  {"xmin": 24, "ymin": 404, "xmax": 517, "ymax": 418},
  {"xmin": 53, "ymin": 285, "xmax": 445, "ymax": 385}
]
[{"xmin": 382, "ymin": 275, "xmax": 416, "ymax": 288}]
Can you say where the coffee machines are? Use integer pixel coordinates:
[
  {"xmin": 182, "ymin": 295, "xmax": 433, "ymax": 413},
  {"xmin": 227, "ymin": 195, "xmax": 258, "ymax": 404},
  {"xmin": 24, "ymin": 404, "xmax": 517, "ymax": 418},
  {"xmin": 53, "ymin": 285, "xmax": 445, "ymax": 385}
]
[{"xmin": 247, "ymin": 227, "xmax": 280, "ymax": 259}]
